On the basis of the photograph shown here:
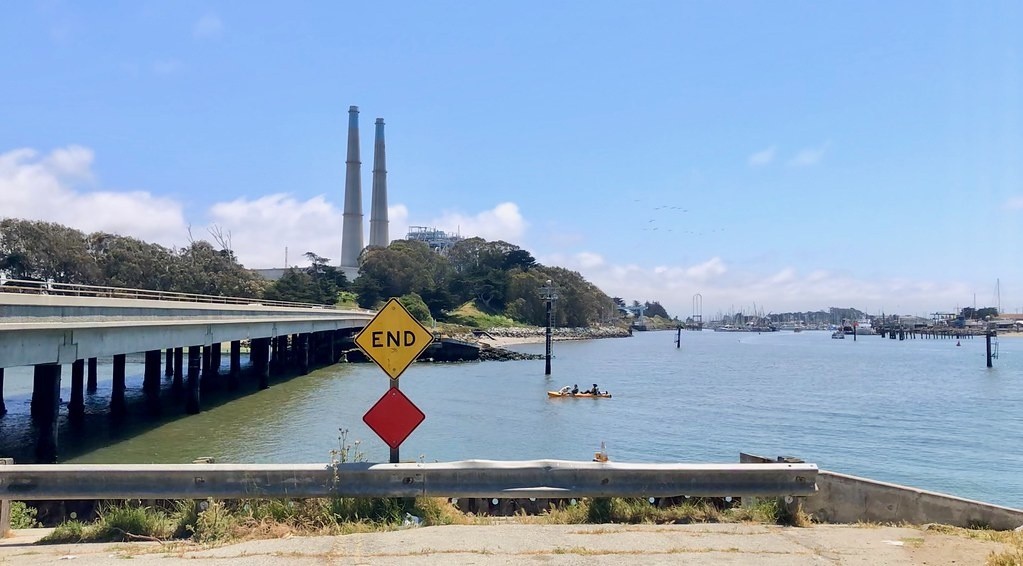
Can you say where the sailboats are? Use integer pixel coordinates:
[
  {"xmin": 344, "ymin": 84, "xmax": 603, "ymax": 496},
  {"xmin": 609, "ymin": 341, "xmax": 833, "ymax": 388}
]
[
  {"xmin": 713, "ymin": 302, "xmax": 777, "ymax": 332},
  {"xmin": 987, "ymin": 278, "xmax": 1013, "ymax": 331}
]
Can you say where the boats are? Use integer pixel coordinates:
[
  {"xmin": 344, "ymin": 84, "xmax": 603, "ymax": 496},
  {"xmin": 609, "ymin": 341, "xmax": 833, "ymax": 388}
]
[
  {"xmin": 855, "ymin": 313, "xmax": 876, "ymax": 335},
  {"xmin": 840, "ymin": 318, "xmax": 855, "ymax": 334},
  {"xmin": 547, "ymin": 386, "xmax": 611, "ymax": 398},
  {"xmin": 831, "ymin": 331, "xmax": 845, "ymax": 339}
]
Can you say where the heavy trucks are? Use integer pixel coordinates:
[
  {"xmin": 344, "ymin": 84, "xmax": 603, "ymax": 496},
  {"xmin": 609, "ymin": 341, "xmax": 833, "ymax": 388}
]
[{"xmin": 950, "ymin": 314, "xmax": 987, "ymax": 328}]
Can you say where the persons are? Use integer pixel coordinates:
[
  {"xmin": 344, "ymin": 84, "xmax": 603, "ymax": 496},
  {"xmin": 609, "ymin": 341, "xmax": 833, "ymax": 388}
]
[
  {"xmin": 559, "ymin": 386, "xmax": 570, "ymax": 394},
  {"xmin": 572, "ymin": 385, "xmax": 578, "ymax": 394},
  {"xmin": 580, "ymin": 384, "xmax": 599, "ymax": 393}
]
